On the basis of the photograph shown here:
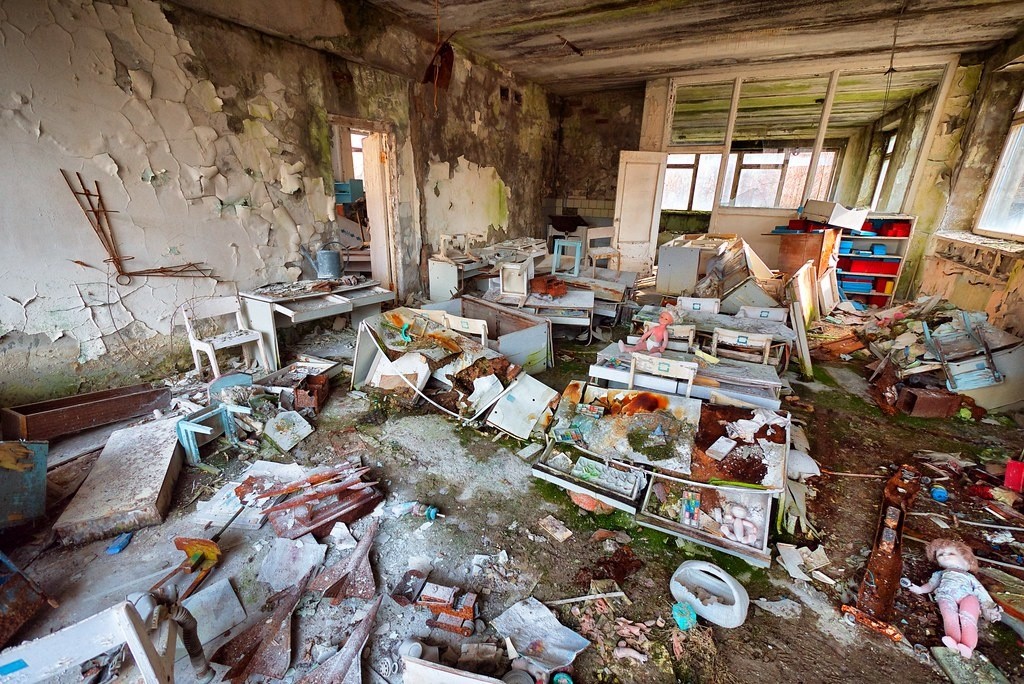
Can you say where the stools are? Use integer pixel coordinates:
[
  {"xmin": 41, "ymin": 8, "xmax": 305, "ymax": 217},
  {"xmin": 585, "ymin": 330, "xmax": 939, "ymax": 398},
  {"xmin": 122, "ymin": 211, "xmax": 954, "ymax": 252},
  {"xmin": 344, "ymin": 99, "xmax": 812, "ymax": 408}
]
[
  {"xmin": 551, "ymin": 238, "xmax": 581, "ymax": 277},
  {"xmin": 175, "ymin": 403, "xmax": 258, "ymax": 475}
]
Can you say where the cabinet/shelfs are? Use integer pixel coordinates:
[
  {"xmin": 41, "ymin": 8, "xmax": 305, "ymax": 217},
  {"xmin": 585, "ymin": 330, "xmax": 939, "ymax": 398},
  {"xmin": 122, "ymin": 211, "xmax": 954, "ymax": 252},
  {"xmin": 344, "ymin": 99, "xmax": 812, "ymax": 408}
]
[
  {"xmin": 823, "ymin": 214, "xmax": 918, "ymax": 307},
  {"xmin": 913, "ymin": 228, "xmax": 1024, "ymax": 333}
]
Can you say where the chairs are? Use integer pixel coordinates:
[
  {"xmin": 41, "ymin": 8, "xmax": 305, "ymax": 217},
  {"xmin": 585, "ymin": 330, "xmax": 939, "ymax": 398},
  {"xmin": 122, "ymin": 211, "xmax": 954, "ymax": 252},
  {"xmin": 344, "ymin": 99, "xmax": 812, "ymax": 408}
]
[
  {"xmin": 628, "ymin": 352, "xmax": 697, "ymax": 399},
  {"xmin": 676, "ymin": 296, "xmax": 720, "ymax": 314},
  {"xmin": 585, "ymin": 227, "xmax": 621, "ymax": 279},
  {"xmin": 442, "ymin": 310, "xmax": 490, "ymax": 349},
  {"xmin": 921, "ymin": 311, "xmax": 1005, "ymax": 393},
  {"xmin": 642, "ymin": 322, "xmax": 697, "ymax": 357},
  {"xmin": 181, "ymin": 295, "xmax": 270, "ymax": 379},
  {"xmin": 738, "ymin": 306, "xmax": 789, "ymax": 320},
  {"xmin": 710, "ymin": 327, "xmax": 773, "ymax": 367},
  {"xmin": 488, "ymin": 258, "xmax": 533, "ymax": 309}
]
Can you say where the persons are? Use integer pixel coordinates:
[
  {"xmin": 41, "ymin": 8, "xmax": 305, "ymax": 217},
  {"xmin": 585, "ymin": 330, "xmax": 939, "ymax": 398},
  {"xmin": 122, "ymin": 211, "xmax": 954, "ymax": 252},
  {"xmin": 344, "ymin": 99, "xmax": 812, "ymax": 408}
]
[
  {"xmin": 907, "ymin": 537, "xmax": 1001, "ymax": 658},
  {"xmin": 352, "ymin": 191, "xmax": 368, "ymax": 228},
  {"xmin": 619, "ymin": 312, "xmax": 674, "ymax": 355}
]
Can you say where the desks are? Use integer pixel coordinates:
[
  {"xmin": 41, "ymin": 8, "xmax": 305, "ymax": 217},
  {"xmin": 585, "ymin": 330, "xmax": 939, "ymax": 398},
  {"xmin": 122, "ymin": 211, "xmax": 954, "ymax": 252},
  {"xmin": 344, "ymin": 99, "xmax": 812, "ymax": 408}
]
[
  {"xmin": 635, "ymin": 304, "xmax": 792, "ymax": 367},
  {"xmin": 238, "ymin": 276, "xmax": 394, "ymax": 370},
  {"xmin": 589, "ymin": 342, "xmax": 781, "ymax": 411},
  {"xmin": 428, "ymin": 239, "xmax": 546, "ymax": 303}
]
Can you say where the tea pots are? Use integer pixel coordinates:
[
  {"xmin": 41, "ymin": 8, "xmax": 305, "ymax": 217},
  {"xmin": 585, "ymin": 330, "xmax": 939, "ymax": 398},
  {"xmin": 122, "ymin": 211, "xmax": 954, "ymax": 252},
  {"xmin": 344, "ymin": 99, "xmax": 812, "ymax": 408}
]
[{"xmin": 300, "ymin": 241, "xmax": 350, "ymax": 280}]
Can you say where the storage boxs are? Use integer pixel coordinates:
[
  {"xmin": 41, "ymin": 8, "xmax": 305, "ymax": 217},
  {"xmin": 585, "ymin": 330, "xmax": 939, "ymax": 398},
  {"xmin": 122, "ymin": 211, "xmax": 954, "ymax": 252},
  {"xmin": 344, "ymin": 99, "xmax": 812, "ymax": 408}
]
[
  {"xmin": 337, "ymin": 216, "xmax": 369, "ymax": 248},
  {"xmin": 425, "ymin": 293, "xmax": 554, "ymax": 375},
  {"xmin": 800, "ymin": 199, "xmax": 869, "ymax": 231}
]
